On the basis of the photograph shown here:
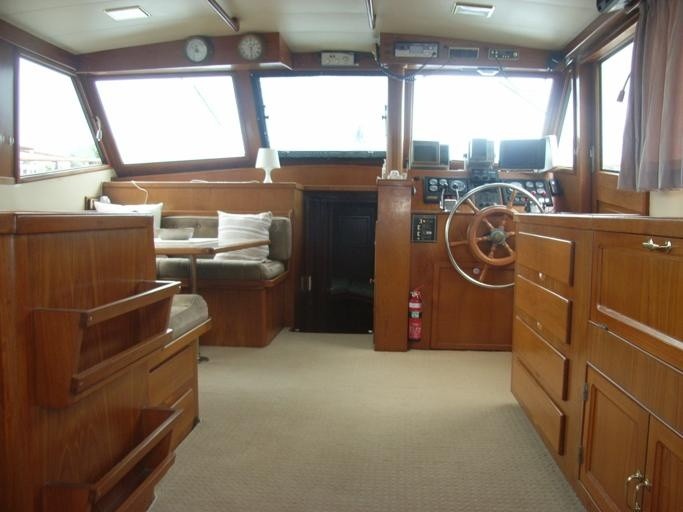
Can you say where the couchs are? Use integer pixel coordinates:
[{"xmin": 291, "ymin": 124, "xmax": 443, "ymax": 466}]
[{"xmin": 94, "ymin": 210, "xmax": 292, "ymax": 348}]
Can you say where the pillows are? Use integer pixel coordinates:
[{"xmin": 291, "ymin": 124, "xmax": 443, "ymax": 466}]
[
  {"xmin": 94, "ymin": 201, "xmax": 163, "ymax": 239},
  {"xmin": 213, "ymin": 211, "xmax": 271, "ymax": 261}
]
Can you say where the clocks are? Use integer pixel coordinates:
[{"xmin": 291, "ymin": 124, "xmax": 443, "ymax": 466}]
[{"xmin": 183, "ymin": 33, "xmax": 263, "ymax": 63}]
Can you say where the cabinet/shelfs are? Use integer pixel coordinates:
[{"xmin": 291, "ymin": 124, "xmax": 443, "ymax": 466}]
[{"xmin": 510, "ymin": 212, "xmax": 683, "ymax": 512}]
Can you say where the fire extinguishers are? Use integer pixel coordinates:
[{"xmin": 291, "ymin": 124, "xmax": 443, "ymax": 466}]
[{"xmin": 409, "ymin": 284, "xmax": 423, "ymax": 341}]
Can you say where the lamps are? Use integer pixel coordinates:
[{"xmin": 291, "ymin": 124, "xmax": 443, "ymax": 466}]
[{"xmin": 256, "ymin": 147, "xmax": 282, "ymax": 182}]
